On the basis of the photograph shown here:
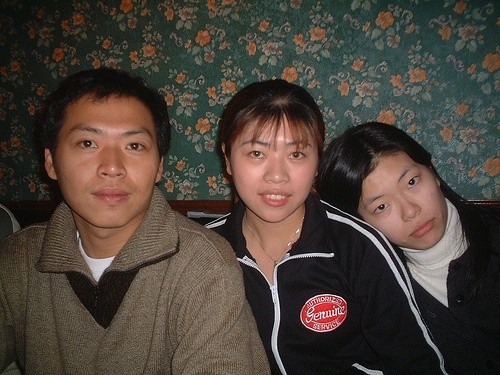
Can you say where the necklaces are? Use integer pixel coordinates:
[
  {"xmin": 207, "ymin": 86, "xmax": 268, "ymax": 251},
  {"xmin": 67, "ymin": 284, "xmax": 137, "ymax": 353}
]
[{"xmin": 243, "ymin": 214, "xmax": 303, "ymax": 267}]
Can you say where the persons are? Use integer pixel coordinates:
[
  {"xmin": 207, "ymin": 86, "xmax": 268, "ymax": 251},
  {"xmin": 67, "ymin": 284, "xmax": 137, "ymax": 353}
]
[
  {"xmin": 204, "ymin": 79, "xmax": 448, "ymax": 375},
  {"xmin": 316, "ymin": 121, "xmax": 500, "ymax": 375},
  {"xmin": 0, "ymin": 66, "xmax": 272, "ymax": 375}
]
